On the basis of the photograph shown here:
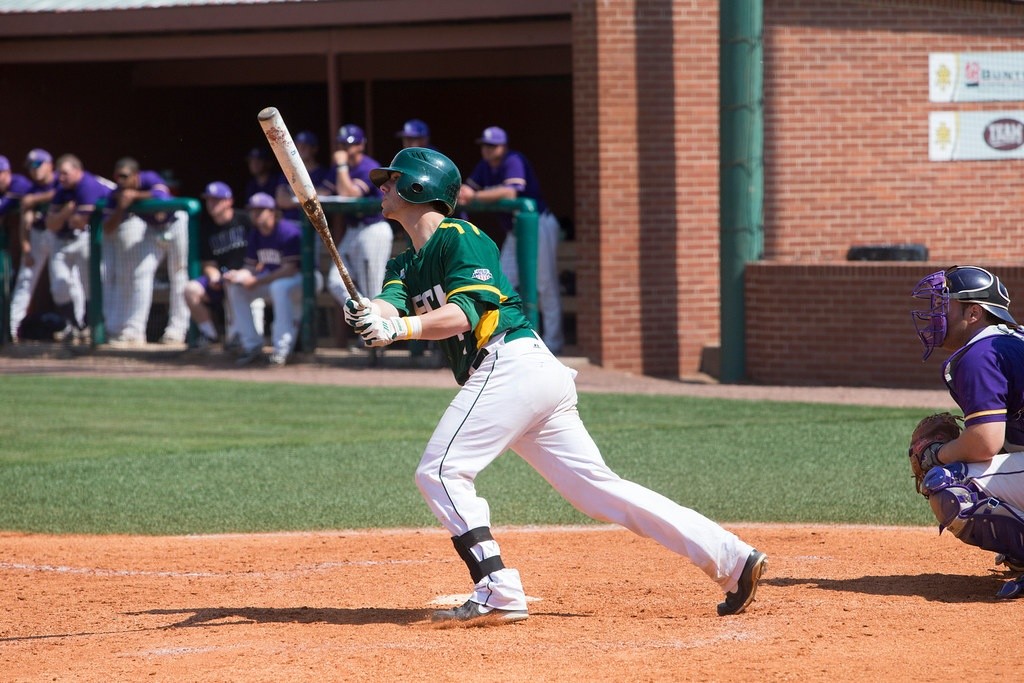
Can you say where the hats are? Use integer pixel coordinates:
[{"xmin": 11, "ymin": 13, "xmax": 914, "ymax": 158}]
[
  {"xmin": 246, "ymin": 146, "xmax": 274, "ymax": 165},
  {"xmin": 336, "ymin": 126, "xmax": 364, "ymax": 144},
  {"xmin": 399, "ymin": 121, "xmax": 430, "ymax": 138},
  {"xmin": 478, "ymin": 126, "xmax": 508, "ymax": 146},
  {"xmin": 0, "ymin": 156, "xmax": 10, "ymax": 171},
  {"xmin": 298, "ymin": 133, "xmax": 319, "ymax": 146},
  {"xmin": 248, "ymin": 193, "xmax": 275, "ymax": 210},
  {"xmin": 201, "ymin": 180, "xmax": 232, "ymax": 199},
  {"xmin": 26, "ymin": 149, "xmax": 51, "ymax": 168}
]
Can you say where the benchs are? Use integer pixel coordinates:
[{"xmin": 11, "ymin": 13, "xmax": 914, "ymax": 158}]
[{"xmin": 150, "ymin": 287, "xmax": 348, "ymax": 348}]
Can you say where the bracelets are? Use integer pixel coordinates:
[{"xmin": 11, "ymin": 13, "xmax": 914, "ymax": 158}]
[{"xmin": 402, "ymin": 316, "xmax": 413, "ymax": 340}]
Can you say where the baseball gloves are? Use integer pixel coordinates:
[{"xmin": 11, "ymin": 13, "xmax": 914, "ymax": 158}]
[{"xmin": 908, "ymin": 411, "xmax": 961, "ymax": 481}]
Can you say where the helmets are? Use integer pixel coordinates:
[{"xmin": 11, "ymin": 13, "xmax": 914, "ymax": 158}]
[
  {"xmin": 368, "ymin": 148, "xmax": 462, "ymax": 216},
  {"xmin": 942, "ymin": 266, "xmax": 1017, "ymax": 325}
]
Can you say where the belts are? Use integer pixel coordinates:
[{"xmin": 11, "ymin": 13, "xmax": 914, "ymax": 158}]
[{"xmin": 465, "ymin": 328, "xmax": 539, "ymax": 379}]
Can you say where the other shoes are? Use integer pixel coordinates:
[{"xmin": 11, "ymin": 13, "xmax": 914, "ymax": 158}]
[
  {"xmin": 1003, "ymin": 555, "xmax": 1024, "ymax": 572},
  {"xmin": 232, "ymin": 352, "xmax": 262, "ymax": 367},
  {"xmin": 185, "ymin": 334, "xmax": 222, "ymax": 350},
  {"xmin": 268, "ymin": 353, "xmax": 283, "ymax": 368},
  {"xmin": 109, "ymin": 335, "xmax": 145, "ymax": 348}
]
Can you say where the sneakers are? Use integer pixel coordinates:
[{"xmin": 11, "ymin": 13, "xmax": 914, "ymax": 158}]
[
  {"xmin": 717, "ymin": 549, "xmax": 766, "ymax": 617},
  {"xmin": 432, "ymin": 601, "xmax": 528, "ymax": 628}
]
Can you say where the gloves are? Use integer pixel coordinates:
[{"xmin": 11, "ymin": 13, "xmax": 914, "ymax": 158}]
[
  {"xmin": 355, "ymin": 316, "xmax": 422, "ymax": 348},
  {"xmin": 918, "ymin": 442, "xmax": 945, "ymax": 471},
  {"xmin": 343, "ymin": 296, "xmax": 399, "ymax": 327}
]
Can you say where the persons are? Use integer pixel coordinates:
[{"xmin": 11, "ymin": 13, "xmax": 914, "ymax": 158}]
[
  {"xmin": 908, "ymin": 266, "xmax": 1024, "ymax": 601},
  {"xmin": 342, "ymin": 147, "xmax": 768, "ymax": 629},
  {"xmin": 457, "ymin": 126, "xmax": 567, "ymax": 353},
  {"xmin": 0, "ymin": 118, "xmax": 469, "ymax": 370}
]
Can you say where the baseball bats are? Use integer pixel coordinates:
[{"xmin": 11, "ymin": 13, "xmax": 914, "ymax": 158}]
[{"xmin": 258, "ymin": 105, "xmax": 362, "ymax": 304}]
